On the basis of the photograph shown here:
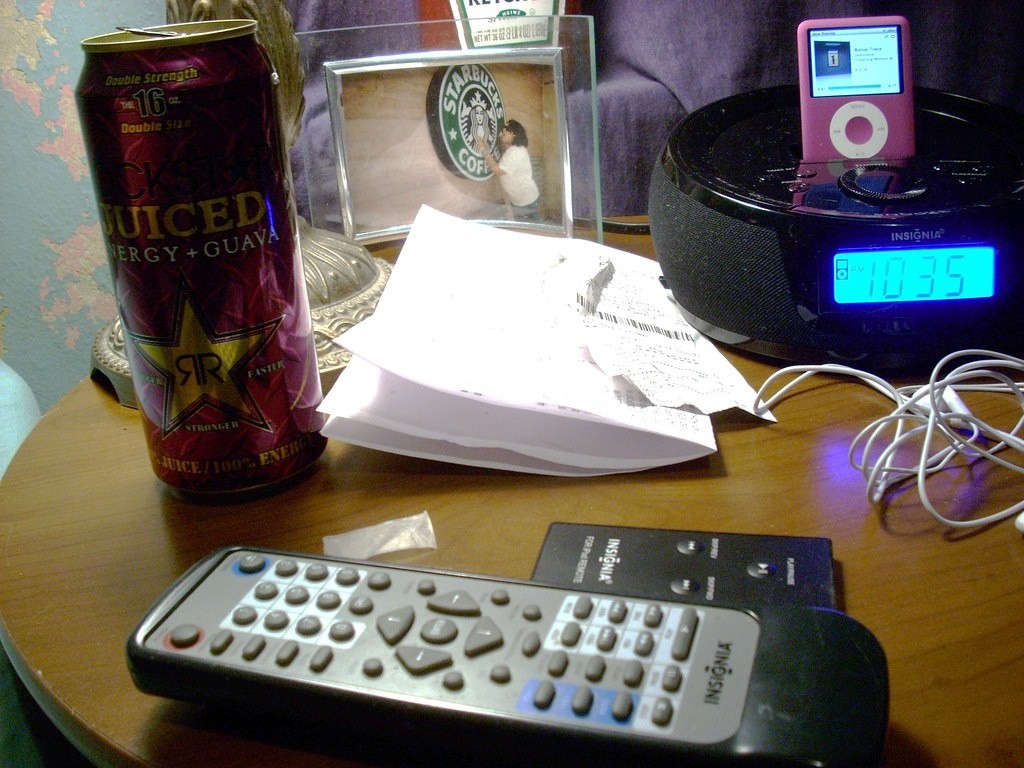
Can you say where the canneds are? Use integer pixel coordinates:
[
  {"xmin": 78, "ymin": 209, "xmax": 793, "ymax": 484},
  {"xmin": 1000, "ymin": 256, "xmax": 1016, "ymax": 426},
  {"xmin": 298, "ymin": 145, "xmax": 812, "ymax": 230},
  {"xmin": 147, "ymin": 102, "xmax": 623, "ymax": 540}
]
[{"xmin": 76, "ymin": 19, "xmax": 328, "ymax": 504}]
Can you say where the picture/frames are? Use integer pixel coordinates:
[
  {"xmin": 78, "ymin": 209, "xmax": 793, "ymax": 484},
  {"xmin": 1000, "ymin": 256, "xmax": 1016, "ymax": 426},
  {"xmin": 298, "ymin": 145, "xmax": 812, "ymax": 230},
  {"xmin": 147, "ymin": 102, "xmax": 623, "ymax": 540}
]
[{"xmin": 294, "ymin": 15, "xmax": 606, "ymax": 253}]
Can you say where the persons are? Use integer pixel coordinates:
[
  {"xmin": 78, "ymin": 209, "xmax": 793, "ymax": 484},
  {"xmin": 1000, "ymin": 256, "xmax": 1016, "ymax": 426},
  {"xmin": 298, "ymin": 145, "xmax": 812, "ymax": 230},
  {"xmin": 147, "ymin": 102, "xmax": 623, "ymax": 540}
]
[{"xmin": 476, "ymin": 120, "xmax": 543, "ymax": 224}]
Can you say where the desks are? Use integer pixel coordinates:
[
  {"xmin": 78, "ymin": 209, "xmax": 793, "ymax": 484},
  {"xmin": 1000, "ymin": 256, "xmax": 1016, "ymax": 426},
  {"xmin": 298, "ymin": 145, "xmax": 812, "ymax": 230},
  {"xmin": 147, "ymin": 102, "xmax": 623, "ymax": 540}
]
[{"xmin": 0, "ymin": 214, "xmax": 1024, "ymax": 768}]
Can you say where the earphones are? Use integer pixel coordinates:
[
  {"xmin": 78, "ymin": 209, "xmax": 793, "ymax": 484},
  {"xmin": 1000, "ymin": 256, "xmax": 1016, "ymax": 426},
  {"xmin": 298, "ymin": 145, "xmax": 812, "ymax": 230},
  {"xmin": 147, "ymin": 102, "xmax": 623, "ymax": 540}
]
[{"xmin": 913, "ymin": 384, "xmax": 973, "ymax": 422}]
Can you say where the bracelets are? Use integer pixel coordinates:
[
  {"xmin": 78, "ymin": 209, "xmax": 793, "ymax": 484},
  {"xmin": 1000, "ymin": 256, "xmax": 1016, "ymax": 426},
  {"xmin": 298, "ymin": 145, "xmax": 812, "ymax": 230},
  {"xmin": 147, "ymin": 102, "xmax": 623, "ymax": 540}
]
[{"xmin": 482, "ymin": 149, "xmax": 490, "ymax": 155}]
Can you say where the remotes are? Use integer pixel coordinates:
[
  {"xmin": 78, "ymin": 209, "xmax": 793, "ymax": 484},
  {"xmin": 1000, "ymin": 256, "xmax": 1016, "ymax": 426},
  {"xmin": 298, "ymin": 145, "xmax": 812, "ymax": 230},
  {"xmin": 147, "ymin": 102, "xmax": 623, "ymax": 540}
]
[{"xmin": 121, "ymin": 544, "xmax": 889, "ymax": 768}]
